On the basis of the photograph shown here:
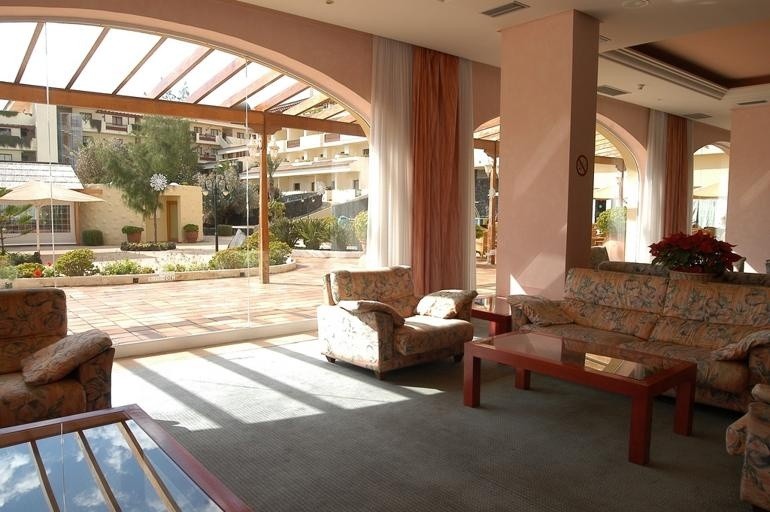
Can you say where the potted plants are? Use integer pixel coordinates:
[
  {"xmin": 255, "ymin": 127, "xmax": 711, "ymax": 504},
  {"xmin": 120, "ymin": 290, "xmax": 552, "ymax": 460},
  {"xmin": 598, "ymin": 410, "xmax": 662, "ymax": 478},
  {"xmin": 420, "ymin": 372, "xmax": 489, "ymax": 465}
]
[
  {"xmin": 121, "ymin": 224, "xmax": 145, "ymax": 245},
  {"xmin": 184, "ymin": 222, "xmax": 199, "ymax": 244}
]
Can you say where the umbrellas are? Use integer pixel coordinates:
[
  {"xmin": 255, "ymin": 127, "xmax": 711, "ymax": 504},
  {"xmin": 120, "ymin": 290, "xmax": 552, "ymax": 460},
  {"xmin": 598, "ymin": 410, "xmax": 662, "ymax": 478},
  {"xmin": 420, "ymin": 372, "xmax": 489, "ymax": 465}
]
[{"xmin": 0, "ymin": 179, "xmax": 107, "ymax": 250}]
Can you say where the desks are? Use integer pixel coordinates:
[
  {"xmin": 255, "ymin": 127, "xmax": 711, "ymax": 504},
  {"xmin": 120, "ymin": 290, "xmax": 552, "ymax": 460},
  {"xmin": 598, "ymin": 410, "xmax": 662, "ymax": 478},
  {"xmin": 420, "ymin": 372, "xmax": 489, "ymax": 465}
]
[{"xmin": 471, "ymin": 292, "xmax": 512, "ymax": 335}]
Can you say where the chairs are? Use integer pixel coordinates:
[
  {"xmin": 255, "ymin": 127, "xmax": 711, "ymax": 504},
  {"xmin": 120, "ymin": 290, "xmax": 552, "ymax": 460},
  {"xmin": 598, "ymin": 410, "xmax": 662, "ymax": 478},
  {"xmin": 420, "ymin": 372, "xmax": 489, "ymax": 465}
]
[
  {"xmin": 315, "ymin": 265, "xmax": 478, "ymax": 384},
  {"xmin": 1, "ymin": 288, "xmax": 120, "ymax": 446}
]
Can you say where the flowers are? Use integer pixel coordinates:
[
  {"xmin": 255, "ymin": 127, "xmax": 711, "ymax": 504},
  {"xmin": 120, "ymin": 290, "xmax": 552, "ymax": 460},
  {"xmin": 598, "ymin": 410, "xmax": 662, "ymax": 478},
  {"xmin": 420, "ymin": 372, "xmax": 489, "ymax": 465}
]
[{"xmin": 649, "ymin": 229, "xmax": 745, "ymax": 276}]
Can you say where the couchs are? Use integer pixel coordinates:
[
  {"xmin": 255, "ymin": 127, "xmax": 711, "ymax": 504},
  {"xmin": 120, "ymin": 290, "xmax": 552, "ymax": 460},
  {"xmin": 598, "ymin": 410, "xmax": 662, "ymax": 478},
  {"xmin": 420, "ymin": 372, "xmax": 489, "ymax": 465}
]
[{"xmin": 509, "ymin": 266, "xmax": 770, "ymax": 415}]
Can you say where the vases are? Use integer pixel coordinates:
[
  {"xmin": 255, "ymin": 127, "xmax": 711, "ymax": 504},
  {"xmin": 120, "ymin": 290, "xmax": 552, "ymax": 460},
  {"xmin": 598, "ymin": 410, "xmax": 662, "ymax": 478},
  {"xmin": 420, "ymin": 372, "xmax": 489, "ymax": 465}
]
[{"xmin": 668, "ymin": 265, "xmax": 716, "ymax": 283}]
